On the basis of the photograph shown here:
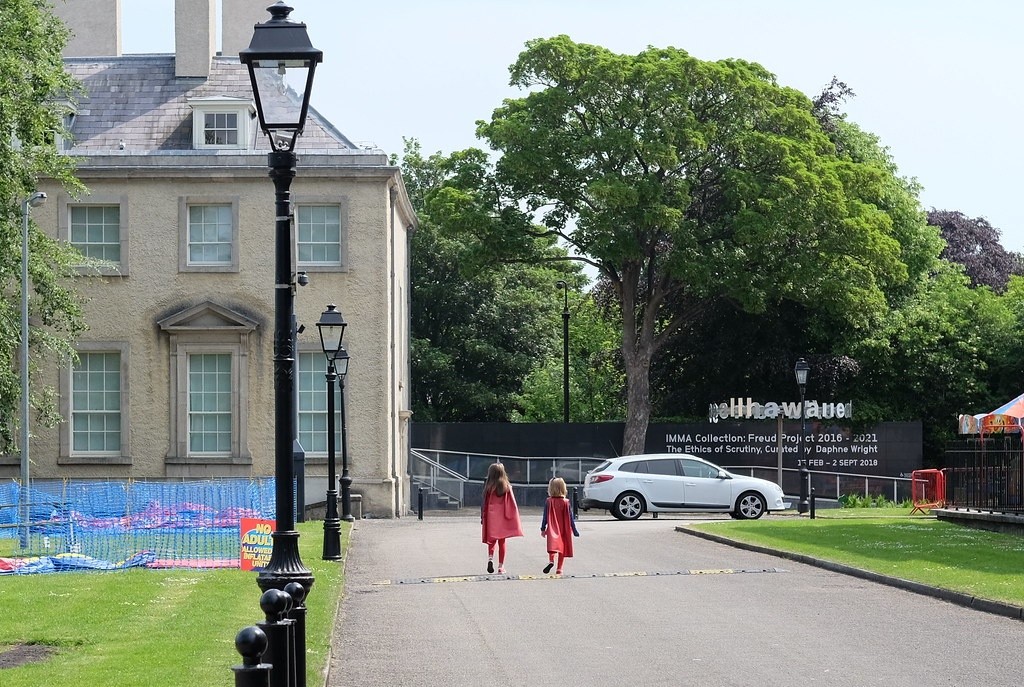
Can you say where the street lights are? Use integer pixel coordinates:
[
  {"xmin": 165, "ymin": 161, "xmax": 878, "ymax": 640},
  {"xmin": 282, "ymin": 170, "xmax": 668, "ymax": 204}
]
[
  {"xmin": 335, "ymin": 345, "xmax": 356, "ymax": 523},
  {"xmin": 238, "ymin": 0, "xmax": 323, "ymax": 685},
  {"xmin": 17, "ymin": 191, "xmax": 48, "ymax": 546},
  {"xmin": 315, "ymin": 305, "xmax": 347, "ymax": 561},
  {"xmin": 557, "ymin": 280, "xmax": 571, "ymax": 424},
  {"xmin": 794, "ymin": 358, "xmax": 810, "ymax": 514}
]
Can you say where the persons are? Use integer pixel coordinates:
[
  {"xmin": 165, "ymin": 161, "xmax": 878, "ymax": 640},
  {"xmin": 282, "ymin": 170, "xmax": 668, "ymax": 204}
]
[
  {"xmin": 480, "ymin": 463, "xmax": 524, "ymax": 572},
  {"xmin": 540, "ymin": 477, "xmax": 579, "ymax": 575}
]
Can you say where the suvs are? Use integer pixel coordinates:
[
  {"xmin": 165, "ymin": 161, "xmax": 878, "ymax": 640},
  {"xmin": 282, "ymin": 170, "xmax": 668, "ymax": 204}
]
[
  {"xmin": 547, "ymin": 457, "xmax": 601, "ymax": 482},
  {"xmin": 579, "ymin": 454, "xmax": 784, "ymax": 521}
]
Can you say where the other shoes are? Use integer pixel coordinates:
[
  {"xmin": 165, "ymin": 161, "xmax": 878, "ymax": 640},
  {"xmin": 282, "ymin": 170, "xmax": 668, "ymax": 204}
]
[
  {"xmin": 487, "ymin": 560, "xmax": 494, "ymax": 573},
  {"xmin": 543, "ymin": 563, "xmax": 554, "ymax": 573},
  {"xmin": 556, "ymin": 570, "xmax": 563, "ymax": 574},
  {"xmin": 498, "ymin": 569, "xmax": 506, "ymax": 575}
]
